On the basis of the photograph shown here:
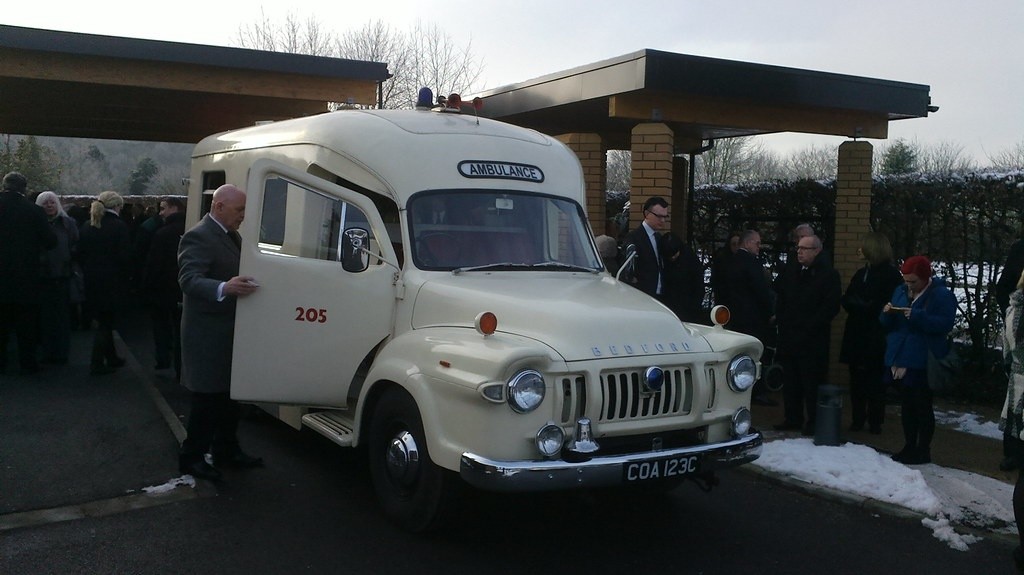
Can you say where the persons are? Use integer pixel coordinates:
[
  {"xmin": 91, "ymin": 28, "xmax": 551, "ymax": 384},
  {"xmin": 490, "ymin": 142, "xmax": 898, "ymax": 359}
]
[
  {"xmin": 878, "ymin": 254, "xmax": 958, "ymax": 465},
  {"xmin": 839, "ymin": 233, "xmax": 906, "ymax": 436},
  {"xmin": 178, "ymin": 184, "xmax": 264, "ymax": 481},
  {"xmin": 594, "ymin": 195, "xmax": 839, "ymax": 438},
  {"xmin": 1, "ymin": 171, "xmax": 187, "ymax": 378},
  {"xmin": 993, "ymin": 239, "xmax": 1024, "ymax": 572}
]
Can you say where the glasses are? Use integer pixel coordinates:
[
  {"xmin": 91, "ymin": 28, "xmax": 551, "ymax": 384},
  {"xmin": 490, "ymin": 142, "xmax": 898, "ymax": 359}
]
[
  {"xmin": 797, "ymin": 245, "xmax": 818, "ymax": 251},
  {"xmin": 648, "ymin": 210, "xmax": 670, "ymax": 220}
]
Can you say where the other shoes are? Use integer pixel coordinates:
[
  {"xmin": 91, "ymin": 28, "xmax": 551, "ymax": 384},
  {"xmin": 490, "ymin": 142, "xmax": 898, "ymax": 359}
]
[
  {"xmin": 848, "ymin": 422, "xmax": 865, "ymax": 431},
  {"xmin": 868, "ymin": 426, "xmax": 880, "ymax": 434},
  {"xmin": 998, "ymin": 454, "xmax": 1023, "ymax": 470},
  {"xmin": 773, "ymin": 421, "xmax": 804, "ymax": 430},
  {"xmin": 89, "ymin": 364, "xmax": 106, "ymax": 375},
  {"xmin": 105, "ymin": 355, "xmax": 123, "ymax": 366},
  {"xmin": 800, "ymin": 428, "xmax": 816, "ymax": 436},
  {"xmin": 892, "ymin": 444, "xmax": 932, "ymax": 465}
]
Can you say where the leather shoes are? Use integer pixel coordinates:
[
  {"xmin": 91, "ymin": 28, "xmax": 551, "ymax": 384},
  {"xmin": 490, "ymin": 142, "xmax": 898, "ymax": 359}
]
[
  {"xmin": 230, "ymin": 453, "xmax": 262, "ymax": 471},
  {"xmin": 181, "ymin": 455, "xmax": 220, "ymax": 480}
]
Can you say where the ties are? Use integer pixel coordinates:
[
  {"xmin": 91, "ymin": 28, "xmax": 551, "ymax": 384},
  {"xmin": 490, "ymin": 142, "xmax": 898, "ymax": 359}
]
[{"xmin": 653, "ymin": 232, "xmax": 664, "ymax": 295}]
